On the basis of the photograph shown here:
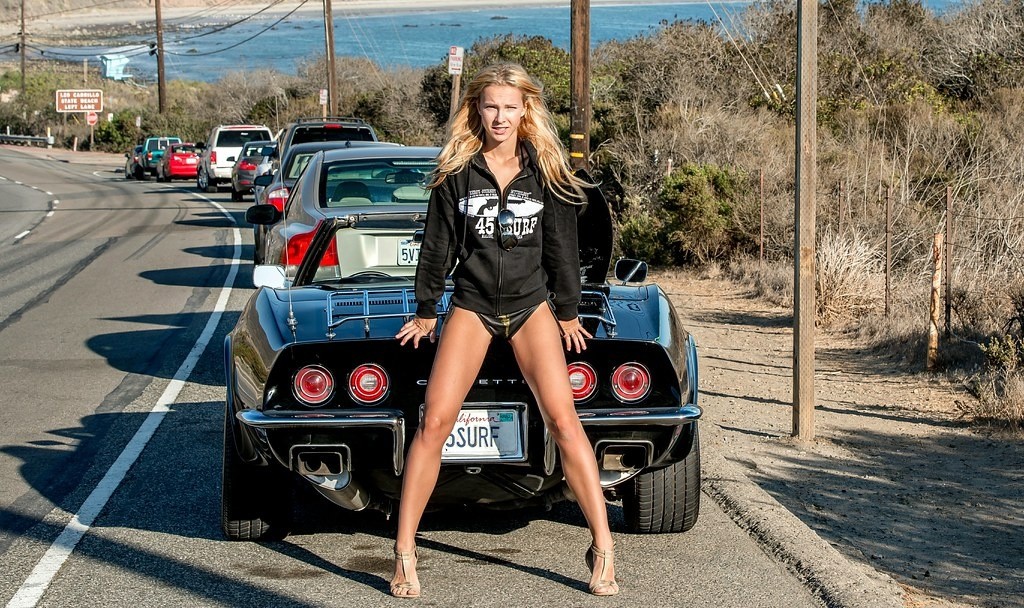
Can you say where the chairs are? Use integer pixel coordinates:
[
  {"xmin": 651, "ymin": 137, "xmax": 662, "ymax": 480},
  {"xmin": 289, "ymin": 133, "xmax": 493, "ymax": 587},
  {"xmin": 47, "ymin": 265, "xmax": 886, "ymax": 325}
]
[
  {"xmin": 331, "ymin": 181, "xmax": 372, "ymax": 203},
  {"xmin": 391, "ymin": 186, "xmax": 432, "ymax": 203}
]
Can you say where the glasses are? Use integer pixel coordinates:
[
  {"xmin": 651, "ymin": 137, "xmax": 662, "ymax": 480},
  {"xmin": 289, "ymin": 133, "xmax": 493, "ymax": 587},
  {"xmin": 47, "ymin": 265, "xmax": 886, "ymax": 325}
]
[{"xmin": 498, "ymin": 208, "xmax": 518, "ymax": 252}]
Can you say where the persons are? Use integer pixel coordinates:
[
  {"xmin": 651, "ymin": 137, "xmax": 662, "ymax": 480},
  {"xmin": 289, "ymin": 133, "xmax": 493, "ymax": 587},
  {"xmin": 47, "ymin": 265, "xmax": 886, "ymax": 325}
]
[{"xmin": 384, "ymin": 63, "xmax": 623, "ymax": 597}]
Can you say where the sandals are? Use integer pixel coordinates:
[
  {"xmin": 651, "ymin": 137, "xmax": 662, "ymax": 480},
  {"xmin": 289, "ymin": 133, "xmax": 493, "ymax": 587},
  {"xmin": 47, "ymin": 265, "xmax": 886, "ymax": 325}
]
[
  {"xmin": 585, "ymin": 542, "xmax": 619, "ymax": 596},
  {"xmin": 390, "ymin": 543, "xmax": 420, "ymax": 598}
]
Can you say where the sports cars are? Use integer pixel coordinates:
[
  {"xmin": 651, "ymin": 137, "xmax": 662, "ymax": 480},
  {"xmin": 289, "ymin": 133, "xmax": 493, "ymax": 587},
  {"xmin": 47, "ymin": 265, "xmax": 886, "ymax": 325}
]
[{"xmin": 222, "ymin": 214, "xmax": 704, "ymax": 544}]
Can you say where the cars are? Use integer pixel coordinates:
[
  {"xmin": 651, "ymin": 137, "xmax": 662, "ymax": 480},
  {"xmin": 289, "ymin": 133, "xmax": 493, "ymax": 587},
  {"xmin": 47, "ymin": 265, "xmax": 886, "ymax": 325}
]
[
  {"xmin": 244, "ymin": 147, "xmax": 446, "ymax": 288},
  {"xmin": 227, "ymin": 142, "xmax": 277, "ymax": 201},
  {"xmin": 155, "ymin": 143, "xmax": 204, "ymax": 182},
  {"xmin": 124, "ymin": 150, "xmax": 138, "ymax": 178},
  {"xmin": 254, "ymin": 140, "xmax": 424, "ymax": 266}
]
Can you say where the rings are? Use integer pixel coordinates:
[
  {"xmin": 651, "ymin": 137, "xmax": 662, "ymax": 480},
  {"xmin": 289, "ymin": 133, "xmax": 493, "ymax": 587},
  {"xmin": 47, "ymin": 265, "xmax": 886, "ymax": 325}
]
[{"xmin": 570, "ymin": 333, "xmax": 576, "ymax": 336}]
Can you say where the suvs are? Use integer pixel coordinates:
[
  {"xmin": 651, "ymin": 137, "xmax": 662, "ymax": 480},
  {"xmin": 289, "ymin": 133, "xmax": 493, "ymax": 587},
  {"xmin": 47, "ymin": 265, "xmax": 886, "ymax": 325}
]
[
  {"xmin": 194, "ymin": 125, "xmax": 274, "ymax": 190},
  {"xmin": 257, "ymin": 117, "xmax": 380, "ymax": 194},
  {"xmin": 134, "ymin": 136, "xmax": 183, "ymax": 180}
]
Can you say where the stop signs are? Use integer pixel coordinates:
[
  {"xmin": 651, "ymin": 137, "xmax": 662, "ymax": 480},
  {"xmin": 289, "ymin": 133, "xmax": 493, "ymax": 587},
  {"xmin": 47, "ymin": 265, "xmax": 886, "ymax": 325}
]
[{"xmin": 87, "ymin": 113, "xmax": 98, "ymax": 126}]
